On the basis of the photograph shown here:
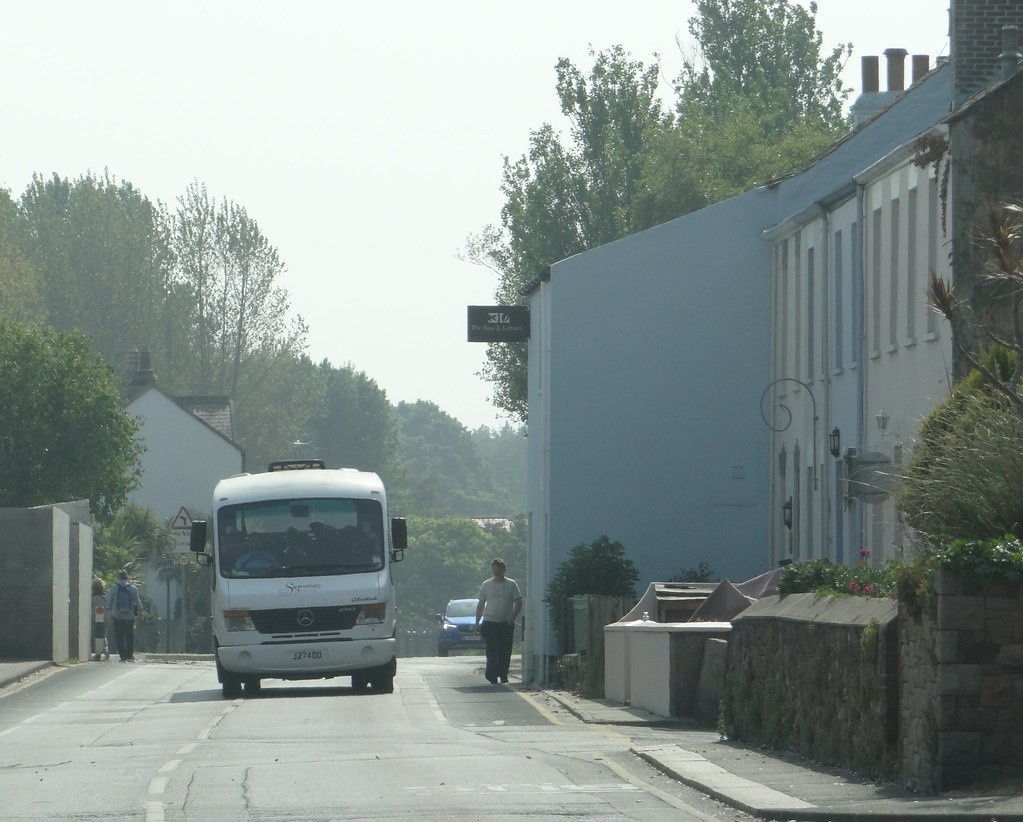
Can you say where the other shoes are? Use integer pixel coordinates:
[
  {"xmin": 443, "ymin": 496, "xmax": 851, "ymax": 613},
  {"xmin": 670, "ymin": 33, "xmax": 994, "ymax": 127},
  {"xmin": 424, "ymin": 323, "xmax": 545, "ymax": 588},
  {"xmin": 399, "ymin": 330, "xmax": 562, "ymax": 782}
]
[
  {"xmin": 102, "ymin": 655, "xmax": 110, "ymax": 662},
  {"xmin": 90, "ymin": 654, "xmax": 101, "ymax": 661},
  {"xmin": 500, "ymin": 675, "xmax": 509, "ymax": 683},
  {"xmin": 485, "ymin": 672, "xmax": 497, "ymax": 684},
  {"xmin": 119, "ymin": 659, "xmax": 126, "ymax": 663}
]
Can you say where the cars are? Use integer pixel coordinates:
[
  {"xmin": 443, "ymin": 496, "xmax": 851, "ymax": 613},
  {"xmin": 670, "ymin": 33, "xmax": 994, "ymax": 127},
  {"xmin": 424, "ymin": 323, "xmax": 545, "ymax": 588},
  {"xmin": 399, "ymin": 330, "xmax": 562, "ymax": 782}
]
[{"xmin": 435, "ymin": 596, "xmax": 487, "ymax": 654}]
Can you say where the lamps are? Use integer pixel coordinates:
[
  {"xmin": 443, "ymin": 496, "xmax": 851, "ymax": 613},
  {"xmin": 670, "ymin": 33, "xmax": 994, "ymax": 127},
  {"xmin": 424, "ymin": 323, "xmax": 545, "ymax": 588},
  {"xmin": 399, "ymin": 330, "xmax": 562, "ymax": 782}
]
[
  {"xmin": 875, "ymin": 407, "xmax": 900, "ymax": 438},
  {"xmin": 829, "ymin": 426, "xmax": 840, "ymax": 459},
  {"xmin": 783, "ymin": 496, "xmax": 792, "ymax": 530}
]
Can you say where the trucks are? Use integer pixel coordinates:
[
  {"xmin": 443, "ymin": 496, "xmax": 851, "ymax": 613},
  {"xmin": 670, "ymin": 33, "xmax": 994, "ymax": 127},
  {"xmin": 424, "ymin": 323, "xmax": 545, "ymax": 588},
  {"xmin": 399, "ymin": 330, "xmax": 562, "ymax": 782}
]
[{"xmin": 189, "ymin": 460, "xmax": 407, "ymax": 696}]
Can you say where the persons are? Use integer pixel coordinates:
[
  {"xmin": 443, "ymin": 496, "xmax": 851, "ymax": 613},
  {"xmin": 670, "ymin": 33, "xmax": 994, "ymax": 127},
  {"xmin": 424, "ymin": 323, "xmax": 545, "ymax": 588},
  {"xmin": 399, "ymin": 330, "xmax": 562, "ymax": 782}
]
[
  {"xmin": 92, "ymin": 569, "xmax": 143, "ymax": 663},
  {"xmin": 235, "ymin": 532, "xmax": 288, "ymax": 570},
  {"xmin": 476, "ymin": 558, "xmax": 522, "ymax": 685}
]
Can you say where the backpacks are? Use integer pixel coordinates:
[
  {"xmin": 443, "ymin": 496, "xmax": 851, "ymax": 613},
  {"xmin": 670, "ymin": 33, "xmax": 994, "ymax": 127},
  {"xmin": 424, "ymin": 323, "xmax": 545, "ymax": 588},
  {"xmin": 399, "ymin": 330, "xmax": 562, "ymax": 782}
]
[{"xmin": 115, "ymin": 582, "xmax": 133, "ymax": 621}]
